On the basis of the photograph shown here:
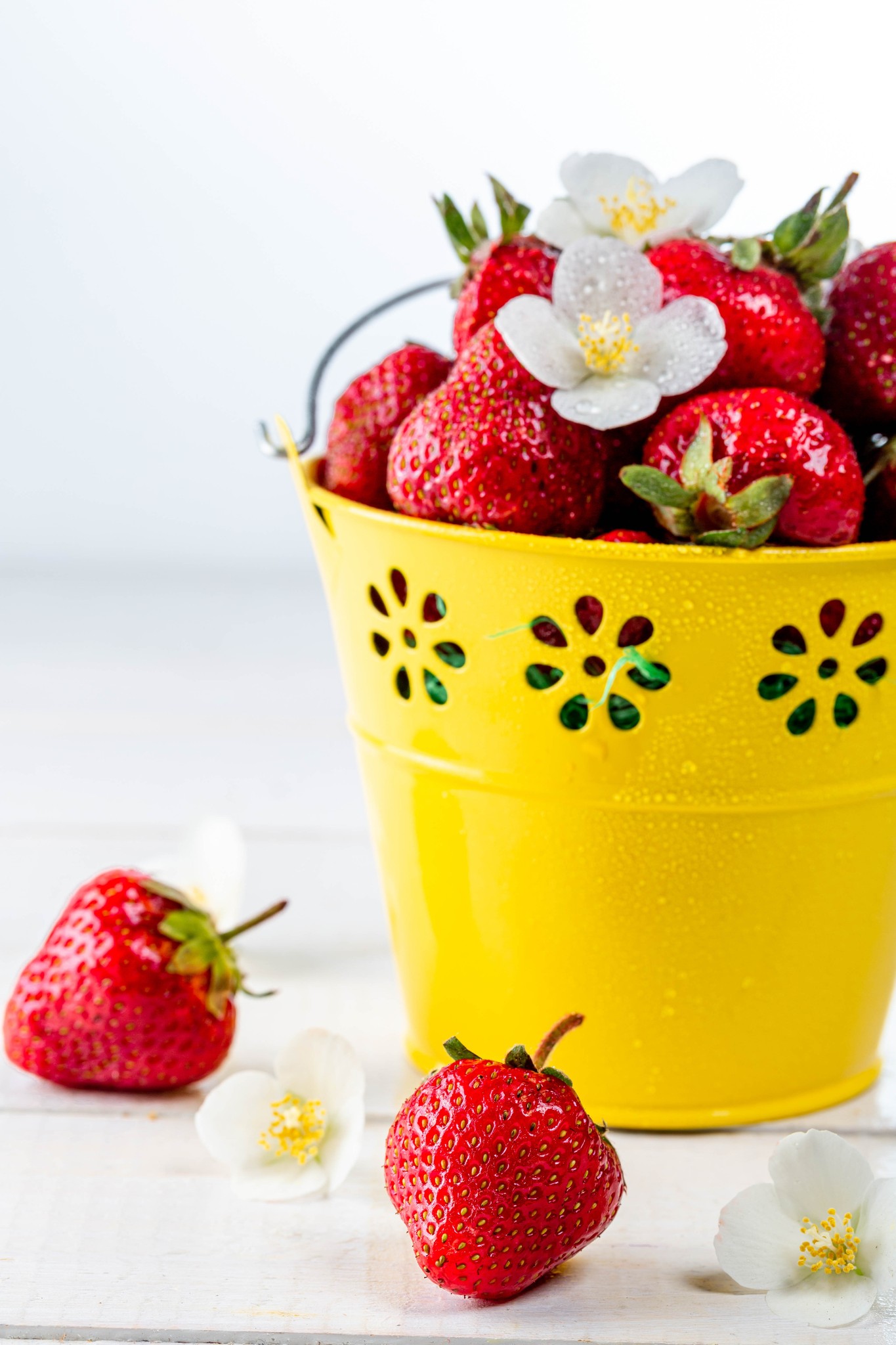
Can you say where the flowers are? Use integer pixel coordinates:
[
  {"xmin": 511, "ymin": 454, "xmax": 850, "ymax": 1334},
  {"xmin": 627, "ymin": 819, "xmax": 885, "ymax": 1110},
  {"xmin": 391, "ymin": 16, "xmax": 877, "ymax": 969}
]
[
  {"xmin": 705, "ymin": 1123, "xmax": 896, "ymax": 1332},
  {"xmin": 491, "ymin": 231, "xmax": 735, "ymax": 439},
  {"xmin": 519, "ymin": 148, "xmax": 750, "ymax": 255},
  {"xmin": 184, "ymin": 1024, "xmax": 377, "ymax": 1209}
]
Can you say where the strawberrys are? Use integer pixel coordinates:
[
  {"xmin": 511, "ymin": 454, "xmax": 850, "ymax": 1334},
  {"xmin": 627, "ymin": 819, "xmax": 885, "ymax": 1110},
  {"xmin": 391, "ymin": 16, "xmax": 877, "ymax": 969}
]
[
  {"xmin": 383, "ymin": 1015, "xmax": 625, "ymax": 1303},
  {"xmin": 314, "ymin": 169, "xmax": 896, "ymax": 732},
  {"xmin": 4, "ymin": 867, "xmax": 290, "ymax": 1092}
]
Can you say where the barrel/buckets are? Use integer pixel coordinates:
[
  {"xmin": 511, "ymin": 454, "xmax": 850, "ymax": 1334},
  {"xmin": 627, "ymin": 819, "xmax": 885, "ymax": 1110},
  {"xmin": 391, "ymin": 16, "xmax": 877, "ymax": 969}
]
[{"xmin": 258, "ymin": 279, "xmax": 896, "ymax": 1132}]
